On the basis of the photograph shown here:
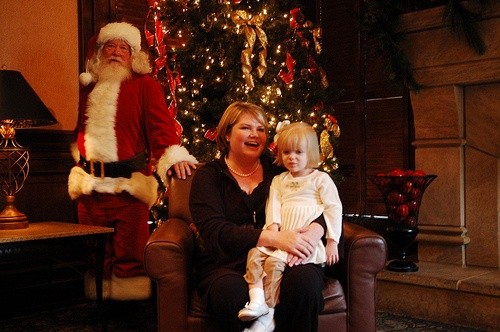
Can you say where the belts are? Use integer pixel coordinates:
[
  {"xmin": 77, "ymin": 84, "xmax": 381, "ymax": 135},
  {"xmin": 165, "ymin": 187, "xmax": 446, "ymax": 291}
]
[{"xmin": 78, "ymin": 149, "xmax": 148, "ymax": 179}]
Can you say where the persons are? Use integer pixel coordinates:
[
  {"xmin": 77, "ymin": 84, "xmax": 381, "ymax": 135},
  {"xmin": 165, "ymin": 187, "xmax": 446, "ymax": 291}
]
[
  {"xmin": 68, "ymin": 23, "xmax": 199, "ymax": 332},
  {"xmin": 189, "ymin": 100, "xmax": 329, "ymax": 332},
  {"xmin": 238, "ymin": 122, "xmax": 343, "ymax": 332}
]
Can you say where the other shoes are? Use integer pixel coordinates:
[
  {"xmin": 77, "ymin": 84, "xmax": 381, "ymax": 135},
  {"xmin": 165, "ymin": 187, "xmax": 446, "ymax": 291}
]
[
  {"xmin": 244, "ymin": 316, "xmax": 276, "ymax": 332},
  {"xmin": 83, "ymin": 302, "xmax": 145, "ymax": 329},
  {"xmin": 238, "ymin": 302, "xmax": 269, "ymax": 322}
]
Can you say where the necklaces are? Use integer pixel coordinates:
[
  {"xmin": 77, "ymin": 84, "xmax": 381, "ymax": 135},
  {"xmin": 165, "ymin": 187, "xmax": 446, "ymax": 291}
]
[{"xmin": 225, "ymin": 155, "xmax": 260, "ymax": 176}]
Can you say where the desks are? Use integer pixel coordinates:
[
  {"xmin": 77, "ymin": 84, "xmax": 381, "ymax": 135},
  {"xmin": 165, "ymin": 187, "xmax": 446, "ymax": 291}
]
[{"xmin": 0, "ymin": 222, "xmax": 115, "ymax": 332}]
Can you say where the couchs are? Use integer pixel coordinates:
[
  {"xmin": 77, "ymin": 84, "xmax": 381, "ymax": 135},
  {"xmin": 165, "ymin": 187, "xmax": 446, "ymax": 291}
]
[{"xmin": 143, "ymin": 163, "xmax": 388, "ymax": 332}]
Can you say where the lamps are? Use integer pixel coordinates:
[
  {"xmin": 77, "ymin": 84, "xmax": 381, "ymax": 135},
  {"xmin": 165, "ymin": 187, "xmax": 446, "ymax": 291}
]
[{"xmin": 0, "ymin": 64, "xmax": 59, "ymax": 230}]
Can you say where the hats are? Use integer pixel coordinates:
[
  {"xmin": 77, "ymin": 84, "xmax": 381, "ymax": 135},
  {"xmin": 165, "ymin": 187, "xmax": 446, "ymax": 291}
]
[{"xmin": 79, "ymin": 20, "xmax": 142, "ymax": 86}]
